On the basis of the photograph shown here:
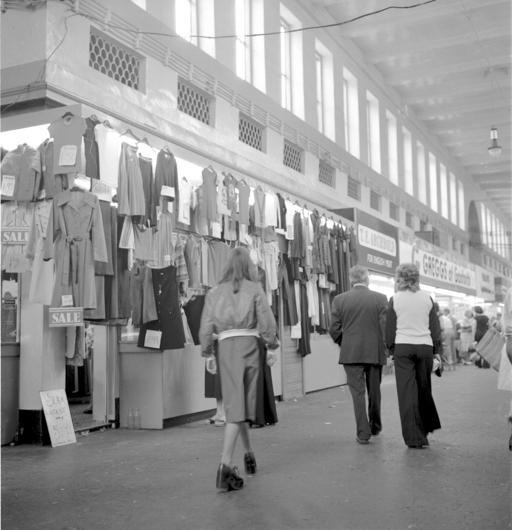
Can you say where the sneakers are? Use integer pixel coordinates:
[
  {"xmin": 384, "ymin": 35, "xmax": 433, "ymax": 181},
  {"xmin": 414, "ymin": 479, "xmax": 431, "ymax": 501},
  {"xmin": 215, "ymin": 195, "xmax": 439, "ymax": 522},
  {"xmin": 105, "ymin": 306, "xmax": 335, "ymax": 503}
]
[{"xmin": 356, "ymin": 438, "xmax": 368, "ymax": 443}]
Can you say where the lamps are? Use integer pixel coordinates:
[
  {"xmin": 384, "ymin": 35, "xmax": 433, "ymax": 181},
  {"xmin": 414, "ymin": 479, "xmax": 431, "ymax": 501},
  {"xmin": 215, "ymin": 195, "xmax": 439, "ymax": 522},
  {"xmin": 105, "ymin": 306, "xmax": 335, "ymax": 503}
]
[{"xmin": 488, "ymin": 128, "xmax": 501, "ymax": 156}]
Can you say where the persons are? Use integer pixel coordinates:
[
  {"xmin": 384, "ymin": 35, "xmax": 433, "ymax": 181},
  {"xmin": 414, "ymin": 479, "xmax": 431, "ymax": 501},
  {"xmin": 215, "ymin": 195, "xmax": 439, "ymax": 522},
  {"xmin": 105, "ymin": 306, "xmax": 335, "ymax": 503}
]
[
  {"xmin": 437, "ymin": 306, "xmax": 502, "ymax": 369},
  {"xmin": 329, "ymin": 264, "xmax": 388, "ymax": 444},
  {"xmin": 384, "ymin": 264, "xmax": 445, "ymax": 450},
  {"xmin": 198, "ymin": 246, "xmax": 280, "ymax": 492},
  {"xmin": 205, "ymin": 331, "xmax": 227, "ymax": 428},
  {"xmin": 503, "ymin": 286, "xmax": 512, "ymax": 450}
]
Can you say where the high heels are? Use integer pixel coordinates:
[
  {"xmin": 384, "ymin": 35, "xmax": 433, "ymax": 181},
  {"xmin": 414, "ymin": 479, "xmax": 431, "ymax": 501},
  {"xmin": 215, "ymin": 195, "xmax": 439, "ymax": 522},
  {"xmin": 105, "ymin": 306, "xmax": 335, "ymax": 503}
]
[
  {"xmin": 216, "ymin": 464, "xmax": 243, "ymax": 490},
  {"xmin": 245, "ymin": 452, "xmax": 256, "ymax": 475}
]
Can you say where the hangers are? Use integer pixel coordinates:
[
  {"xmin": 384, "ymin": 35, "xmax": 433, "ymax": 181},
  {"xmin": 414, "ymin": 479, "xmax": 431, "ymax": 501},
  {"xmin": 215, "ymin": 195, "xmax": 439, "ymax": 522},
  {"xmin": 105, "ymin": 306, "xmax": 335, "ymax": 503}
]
[{"xmin": 55, "ymin": 111, "xmax": 345, "ymax": 225}]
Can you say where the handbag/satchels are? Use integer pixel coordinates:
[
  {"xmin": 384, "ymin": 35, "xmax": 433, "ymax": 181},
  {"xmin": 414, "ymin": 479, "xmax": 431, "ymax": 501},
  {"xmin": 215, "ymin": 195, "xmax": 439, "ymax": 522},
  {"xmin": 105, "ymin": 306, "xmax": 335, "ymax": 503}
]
[
  {"xmin": 476, "ymin": 321, "xmax": 504, "ymax": 372},
  {"xmin": 434, "ymin": 357, "xmax": 442, "ymax": 376}
]
